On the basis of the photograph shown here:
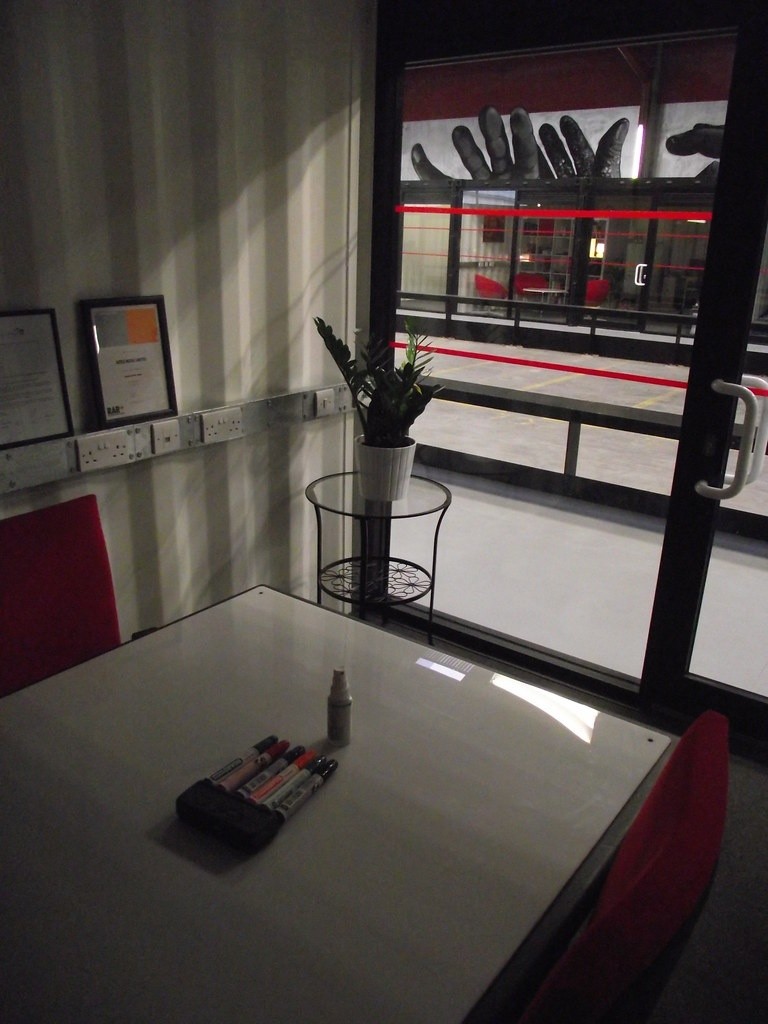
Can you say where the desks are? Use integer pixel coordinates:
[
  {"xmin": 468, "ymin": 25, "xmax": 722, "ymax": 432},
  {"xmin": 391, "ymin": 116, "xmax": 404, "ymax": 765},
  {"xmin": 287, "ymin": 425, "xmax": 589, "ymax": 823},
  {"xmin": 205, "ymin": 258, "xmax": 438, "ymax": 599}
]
[
  {"xmin": 523, "ymin": 287, "xmax": 568, "ymax": 304},
  {"xmin": 0, "ymin": 584, "xmax": 680, "ymax": 1023}
]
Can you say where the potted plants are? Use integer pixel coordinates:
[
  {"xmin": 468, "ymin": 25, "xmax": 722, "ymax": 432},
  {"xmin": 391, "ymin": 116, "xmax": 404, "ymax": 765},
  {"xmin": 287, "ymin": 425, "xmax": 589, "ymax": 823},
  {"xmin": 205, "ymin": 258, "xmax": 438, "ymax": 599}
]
[{"xmin": 312, "ymin": 313, "xmax": 447, "ymax": 502}]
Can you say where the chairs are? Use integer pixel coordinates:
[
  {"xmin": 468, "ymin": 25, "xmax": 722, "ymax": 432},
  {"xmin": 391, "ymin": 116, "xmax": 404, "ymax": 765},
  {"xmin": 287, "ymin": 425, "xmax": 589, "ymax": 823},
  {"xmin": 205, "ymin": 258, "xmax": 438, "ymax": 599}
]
[
  {"xmin": 475, "ymin": 274, "xmax": 509, "ymax": 300},
  {"xmin": 585, "ymin": 279, "xmax": 610, "ymax": 307},
  {"xmin": 515, "ymin": 272, "xmax": 549, "ymax": 296},
  {"xmin": 0, "ymin": 494, "xmax": 120, "ymax": 702},
  {"xmin": 528, "ymin": 709, "xmax": 731, "ymax": 1022}
]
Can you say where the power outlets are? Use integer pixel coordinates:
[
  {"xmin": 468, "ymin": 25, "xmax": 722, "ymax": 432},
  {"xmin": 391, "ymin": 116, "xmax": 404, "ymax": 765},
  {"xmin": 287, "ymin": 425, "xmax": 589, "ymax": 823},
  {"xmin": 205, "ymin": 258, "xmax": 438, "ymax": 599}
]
[
  {"xmin": 76, "ymin": 429, "xmax": 135, "ymax": 472},
  {"xmin": 201, "ymin": 407, "xmax": 245, "ymax": 444}
]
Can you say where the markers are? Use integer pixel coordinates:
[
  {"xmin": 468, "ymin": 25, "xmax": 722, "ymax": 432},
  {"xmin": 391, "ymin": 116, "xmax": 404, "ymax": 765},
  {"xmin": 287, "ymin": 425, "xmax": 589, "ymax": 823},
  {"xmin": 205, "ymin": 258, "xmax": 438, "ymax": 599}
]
[{"xmin": 181, "ymin": 731, "xmax": 337, "ymax": 847}]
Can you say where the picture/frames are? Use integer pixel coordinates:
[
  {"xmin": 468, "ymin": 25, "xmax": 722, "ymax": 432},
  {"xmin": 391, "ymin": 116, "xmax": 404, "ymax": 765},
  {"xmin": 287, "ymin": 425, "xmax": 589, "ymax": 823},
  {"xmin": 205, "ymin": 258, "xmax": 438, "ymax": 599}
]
[
  {"xmin": 0, "ymin": 307, "xmax": 75, "ymax": 449},
  {"xmin": 81, "ymin": 295, "xmax": 178, "ymax": 426}
]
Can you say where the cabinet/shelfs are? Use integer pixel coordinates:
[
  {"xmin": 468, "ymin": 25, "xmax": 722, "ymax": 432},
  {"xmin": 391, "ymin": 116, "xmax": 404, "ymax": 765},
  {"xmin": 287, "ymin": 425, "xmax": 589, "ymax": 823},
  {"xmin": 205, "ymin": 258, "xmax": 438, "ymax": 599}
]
[{"xmin": 304, "ymin": 471, "xmax": 452, "ymax": 647}]
